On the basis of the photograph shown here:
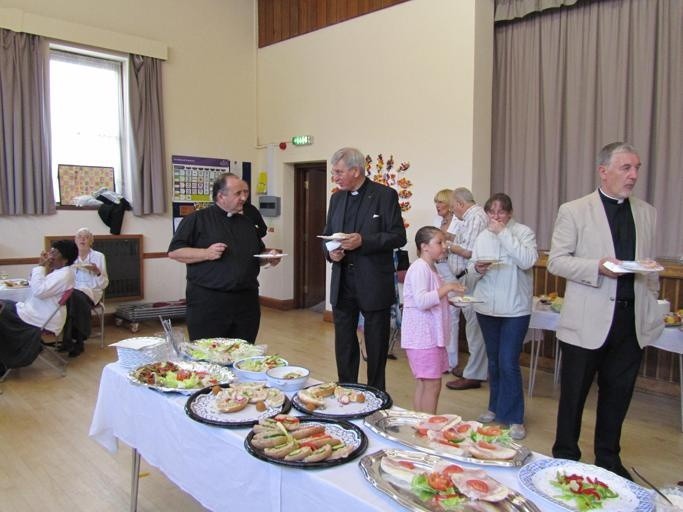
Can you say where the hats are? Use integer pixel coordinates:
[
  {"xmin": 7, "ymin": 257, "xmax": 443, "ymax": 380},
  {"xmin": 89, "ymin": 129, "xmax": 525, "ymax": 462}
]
[{"xmin": 51, "ymin": 241, "xmax": 78, "ymax": 265}]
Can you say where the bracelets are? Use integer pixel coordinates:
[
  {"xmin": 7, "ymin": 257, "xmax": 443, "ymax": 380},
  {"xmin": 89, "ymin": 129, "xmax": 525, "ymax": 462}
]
[{"xmin": 447, "ymin": 242, "xmax": 453, "ymax": 254}]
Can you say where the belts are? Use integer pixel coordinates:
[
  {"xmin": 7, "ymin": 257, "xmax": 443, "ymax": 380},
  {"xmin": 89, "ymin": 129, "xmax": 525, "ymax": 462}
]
[{"xmin": 456, "ymin": 269, "xmax": 468, "ymax": 279}]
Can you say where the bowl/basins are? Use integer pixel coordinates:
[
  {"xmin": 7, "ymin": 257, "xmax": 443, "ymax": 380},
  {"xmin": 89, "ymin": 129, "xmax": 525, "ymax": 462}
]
[
  {"xmin": 233, "ymin": 356, "xmax": 289, "ymax": 380},
  {"xmin": 655, "ymin": 484, "xmax": 683, "ymax": 512},
  {"xmin": 266, "ymin": 365, "xmax": 310, "ymax": 392}
]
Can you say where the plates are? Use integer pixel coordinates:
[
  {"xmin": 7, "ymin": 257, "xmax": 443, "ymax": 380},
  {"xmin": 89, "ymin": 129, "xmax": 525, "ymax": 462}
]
[
  {"xmin": 72, "ymin": 263, "xmax": 92, "ymax": 267},
  {"xmin": 291, "ymin": 383, "xmax": 394, "ymax": 420},
  {"xmin": 364, "ymin": 408, "xmax": 532, "ymax": 467},
  {"xmin": 358, "ymin": 448, "xmax": 541, "ymax": 512},
  {"xmin": 116, "ymin": 336, "xmax": 166, "ymax": 371},
  {"xmin": 184, "ymin": 383, "xmax": 292, "ymax": 428},
  {"xmin": 621, "ymin": 260, "xmax": 665, "ymax": 273},
  {"xmin": 178, "ymin": 337, "xmax": 266, "ymax": 366},
  {"xmin": 316, "ymin": 235, "xmax": 349, "ymax": 240},
  {"xmin": 518, "ymin": 458, "xmax": 656, "ymax": 512},
  {"xmin": 449, "ymin": 297, "xmax": 485, "ymax": 303},
  {"xmin": 253, "ymin": 253, "xmax": 288, "ymax": 258},
  {"xmin": 126, "ymin": 361, "xmax": 235, "ymax": 397},
  {"xmin": 471, "ymin": 258, "xmax": 500, "ymax": 265},
  {"xmin": 244, "ymin": 416, "xmax": 370, "ymax": 470}
]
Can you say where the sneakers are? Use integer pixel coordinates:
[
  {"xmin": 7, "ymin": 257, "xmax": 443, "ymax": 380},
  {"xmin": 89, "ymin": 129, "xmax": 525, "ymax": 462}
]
[
  {"xmin": 476, "ymin": 410, "xmax": 496, "ymax": 423},
  {"xmin": 54, "ymin": 341, "xmax": 84, "ymax": 357},
  {"xmin": 510, "ymin": 424, "xmax": 526, "ymax": 439}
]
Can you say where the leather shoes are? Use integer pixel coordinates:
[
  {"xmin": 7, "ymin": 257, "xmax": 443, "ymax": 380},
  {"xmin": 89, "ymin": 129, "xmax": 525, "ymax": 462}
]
[
  {"xmin": 607, "ymin": 463, "xmax": 633, "ymax": 482},
  {"xmin": 446, "ymin": 367, "xmax": 480, "ymax": 390}
]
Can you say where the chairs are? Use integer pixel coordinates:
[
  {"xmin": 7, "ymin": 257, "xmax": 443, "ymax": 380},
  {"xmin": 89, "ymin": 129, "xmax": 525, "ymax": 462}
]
[
  {"xmin": 0, "ymin": 287, "xmax": 76, "ymax": 382},
  {"xmin": 89, "ymin": 288, "xmax": 107, "ymax": 349}
]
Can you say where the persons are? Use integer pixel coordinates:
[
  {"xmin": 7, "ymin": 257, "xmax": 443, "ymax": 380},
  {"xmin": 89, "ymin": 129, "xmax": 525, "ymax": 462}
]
[
  {"xmin": 444, "ymin": 187, "xmax": 489, "ymax": 390},
  {"xmin": 321, "ymin": 148, "xmax": 407, "ymax": 391},
  {"xmin": 547, "ymin": 142, "xmax": 665, "ymax": 481},
  {"xmin": 167, "ymin": 173, "xmax": 281, "ymax": 344},
  {"xmin": 0, "ymin": 239, "xmax": 79, "ymax": 384},
  {"xmin": 401, "ymin": 227, "xmax": 468, "ymax": 414},
  {"xmin": 56, "ymin": 229, "xmax": 109, "ymax": 357},
  {"xmin": 468, "ymin": 193, "xmax": 539, "ymax": 440},
  {"xmin": 434, "ymin": 189, "xmax": 463, "ymax": 374},
  {"xmin": 239, "ymin": 179, "xmax": 267, "ymax": 238}
]
